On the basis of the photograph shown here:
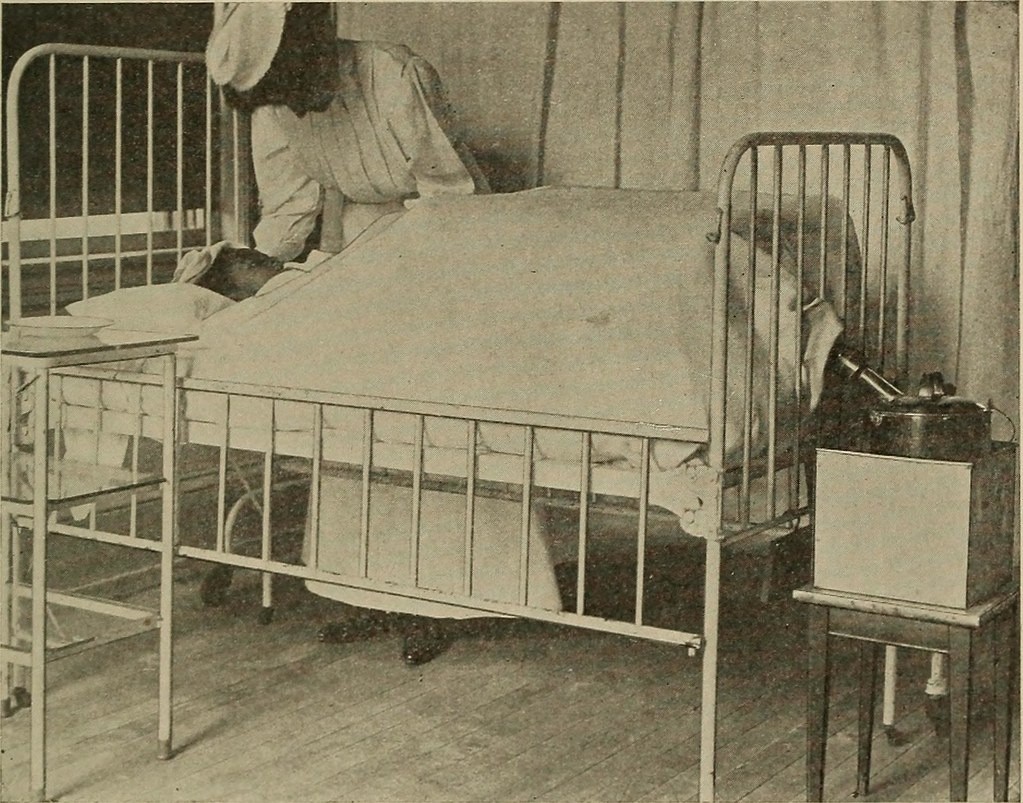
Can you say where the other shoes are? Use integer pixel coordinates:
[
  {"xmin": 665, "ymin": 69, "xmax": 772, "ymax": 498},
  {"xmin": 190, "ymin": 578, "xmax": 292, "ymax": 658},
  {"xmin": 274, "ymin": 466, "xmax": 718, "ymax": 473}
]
[
  {"xmin": 317, "ymin": 614, "xmax": 381, "ymax": 646},
  {"xmin": 400, "ymin": 626, "xmax": 459, "ymax": 665}
]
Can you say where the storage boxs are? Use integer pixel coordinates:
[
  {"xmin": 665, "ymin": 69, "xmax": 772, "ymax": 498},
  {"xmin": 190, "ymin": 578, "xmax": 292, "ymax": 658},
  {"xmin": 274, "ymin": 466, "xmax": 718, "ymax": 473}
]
[{"xmin": 809, "ymin": 444, "xmax": 1017, "ymax": 610}]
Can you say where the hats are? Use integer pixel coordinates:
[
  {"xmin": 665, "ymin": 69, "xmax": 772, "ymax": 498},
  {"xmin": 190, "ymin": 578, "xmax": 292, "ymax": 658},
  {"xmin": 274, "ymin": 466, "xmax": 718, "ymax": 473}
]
[{"xmin": 206, "ymin": 3, "xmax": 292, "ymax": 91}]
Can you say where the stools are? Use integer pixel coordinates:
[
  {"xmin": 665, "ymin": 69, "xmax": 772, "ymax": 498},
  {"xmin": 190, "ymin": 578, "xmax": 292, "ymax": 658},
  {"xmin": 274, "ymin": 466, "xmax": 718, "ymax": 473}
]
[{"xmin": 792, "ymin": 581, "xmax": 1018, "ymax": 802}]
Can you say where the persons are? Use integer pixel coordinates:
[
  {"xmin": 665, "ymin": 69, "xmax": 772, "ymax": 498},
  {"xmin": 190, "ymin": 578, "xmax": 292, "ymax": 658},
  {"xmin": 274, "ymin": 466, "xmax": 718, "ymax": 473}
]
[
  {"xmin": 201, "ymin": 2, "xmax": 596, "ymax": 664},
  {"xmin": 198, "ymin": 244, "xmax": 331, "ymax": 303}
]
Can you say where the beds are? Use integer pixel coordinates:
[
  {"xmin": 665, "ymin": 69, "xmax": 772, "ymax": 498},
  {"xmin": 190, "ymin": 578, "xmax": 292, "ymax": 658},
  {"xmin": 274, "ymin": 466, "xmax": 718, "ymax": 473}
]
[{"xmin": 3, "ymin": 42, "xmax": 913, "ymax": 802}]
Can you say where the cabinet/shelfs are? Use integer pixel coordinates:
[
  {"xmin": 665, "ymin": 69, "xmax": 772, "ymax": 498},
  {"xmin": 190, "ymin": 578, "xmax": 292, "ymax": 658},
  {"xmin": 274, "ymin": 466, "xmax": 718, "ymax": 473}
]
[{"xmin": 4, "ymin": 333, "xmax": 193, "ymax": 799}]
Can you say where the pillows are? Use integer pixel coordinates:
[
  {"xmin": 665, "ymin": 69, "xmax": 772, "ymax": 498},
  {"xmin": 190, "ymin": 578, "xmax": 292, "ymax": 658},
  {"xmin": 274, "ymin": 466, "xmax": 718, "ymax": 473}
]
[{"xmin": 66, "ymin": 249, "xmax": 324, "ymax": 358}]
